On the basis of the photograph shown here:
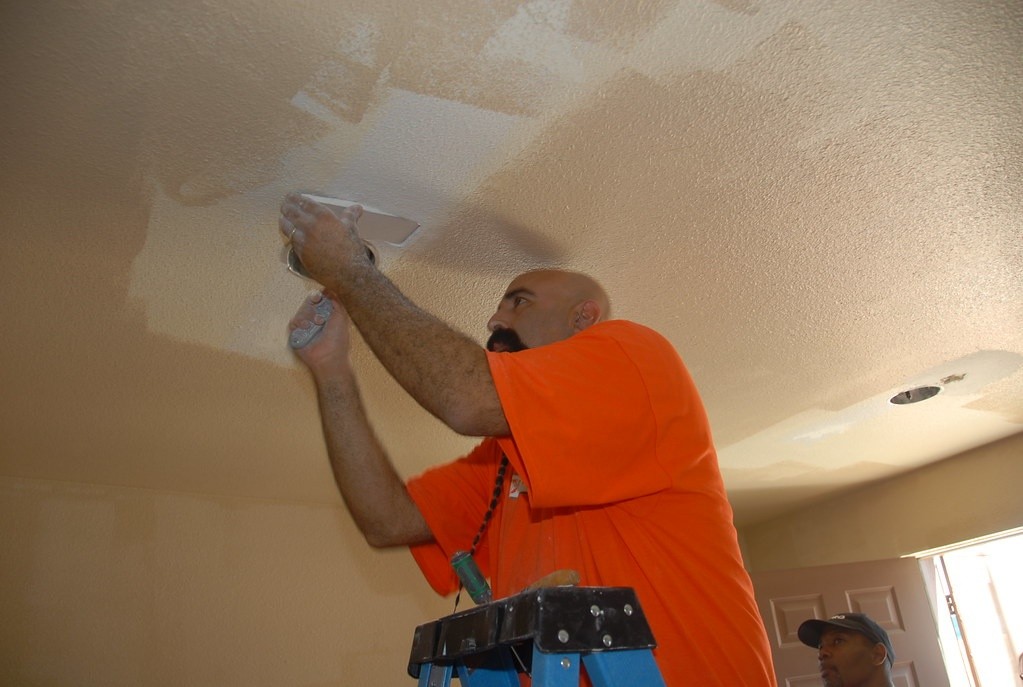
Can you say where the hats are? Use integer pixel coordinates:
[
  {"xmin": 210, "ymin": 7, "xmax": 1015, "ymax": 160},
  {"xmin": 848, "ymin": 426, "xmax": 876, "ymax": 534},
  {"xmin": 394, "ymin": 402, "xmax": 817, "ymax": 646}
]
[{"xmin": 797, "ymin": 612, "xmax": 896, "ymax": 669}]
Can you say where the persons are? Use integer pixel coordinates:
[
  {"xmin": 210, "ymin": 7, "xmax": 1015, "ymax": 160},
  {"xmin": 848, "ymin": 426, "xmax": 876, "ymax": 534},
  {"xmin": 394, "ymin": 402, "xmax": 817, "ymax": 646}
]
[
  {"xmin": 280, "ymin": 191, "xmax": 778, "ymax": 687},
  {"xmin": 798, "ymin": 612, "xmax": 895, "ymax": 687}
]
